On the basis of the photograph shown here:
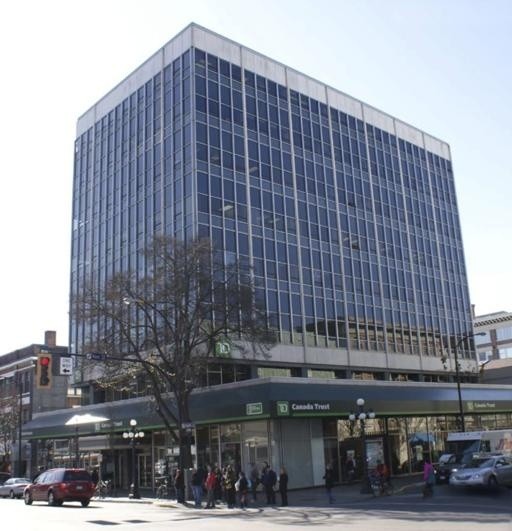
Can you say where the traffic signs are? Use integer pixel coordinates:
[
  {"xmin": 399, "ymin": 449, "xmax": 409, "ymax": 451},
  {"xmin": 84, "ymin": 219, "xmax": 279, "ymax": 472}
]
[
  {"xmin": 60, "ymin": 358, "xmax": 74, "ymax": 376},
  {"xmin": 87, "ymin": 353, "xmax": 108, "ymax": 362}
]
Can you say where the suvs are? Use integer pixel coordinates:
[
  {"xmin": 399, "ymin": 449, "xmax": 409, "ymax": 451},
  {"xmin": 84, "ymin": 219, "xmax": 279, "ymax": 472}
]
[{"xmin": 23, "ymin": 468, "xmax": 97, "ymax": 509}]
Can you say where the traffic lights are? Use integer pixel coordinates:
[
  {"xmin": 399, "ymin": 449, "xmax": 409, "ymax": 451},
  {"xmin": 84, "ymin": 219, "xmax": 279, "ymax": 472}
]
[{"xmin": 37, "ymin": 353, "xmax": 52, "ymax": 389}]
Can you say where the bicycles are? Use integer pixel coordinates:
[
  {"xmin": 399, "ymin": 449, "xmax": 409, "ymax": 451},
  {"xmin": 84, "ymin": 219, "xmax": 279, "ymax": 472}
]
[
  {"xmin": 367, "ymin": 474, "xmax": 394, "ymax": 499},
  {"xmin": 96, "ymin": 479, "xmax": 112, "ymax": 498}
]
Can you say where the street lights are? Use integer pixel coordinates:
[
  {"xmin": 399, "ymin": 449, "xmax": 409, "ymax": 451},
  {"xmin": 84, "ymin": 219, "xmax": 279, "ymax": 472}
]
[
  {"xmin": 453, "ymin": 333, "xmax": 487, "ymax": 432},
  {"xmin": 122, "ymin": 420, "xmax": 146, "ymax": 500},
  {"xmin": 349, "ymin": 398, "xmax": 375, "ymax": 479},
  {"xmin": 1, "ymin": 374, "xmax": 23, "ymax": 477}
]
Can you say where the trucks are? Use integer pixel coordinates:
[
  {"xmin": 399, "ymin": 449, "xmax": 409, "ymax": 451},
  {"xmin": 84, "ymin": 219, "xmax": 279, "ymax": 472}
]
[
  {"xmin": 339, "ymin": 437, "xmax": 402, "ymax": 471},
  {"xmin": 446, "ymin": 430, "xmax": 511, "ymax": 463}
]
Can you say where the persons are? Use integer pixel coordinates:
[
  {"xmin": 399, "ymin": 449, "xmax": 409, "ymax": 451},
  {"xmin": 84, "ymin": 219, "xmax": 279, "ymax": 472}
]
[
  {"xmin": 324, "ymin": 463, "xmax": 338, "ymax": 505},
  {"xmin": 91, "ymin": 469, "xmax": 99, "ymax": 486},
  {"xmin": 278, "ymin": 464, "xmax": 288, "ymax": 507},
  {"xmin": 345, "ymin": 457, "xmax": 354, "ymax": 485},
  {"xmin": 174, "ymin": 461, "xmax": 278, "ymax": 510},
  {"xmin": 376, "ymin": 460, "xmax": 389, "ymax": 493},
  {"xmin": 422, "ymin": 458, "xmax": 434, "ymax": 499},
  {"xmin": 167, "ymin": 517, "xmax": 170, "ymax": 518}
]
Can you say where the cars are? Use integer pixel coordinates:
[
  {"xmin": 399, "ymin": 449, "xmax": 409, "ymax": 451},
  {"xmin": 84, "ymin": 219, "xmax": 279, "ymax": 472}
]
[
  {"xmin": 0, "ymin": 478, "xmax": 32, "ymax": 500},
  {"xmin": 433, "ymin": 453, "xmax": 467, "ymax": 482},
  {"xmin": 448, "ymin": 456, "xmax": 511, "ymax": 494}
]
